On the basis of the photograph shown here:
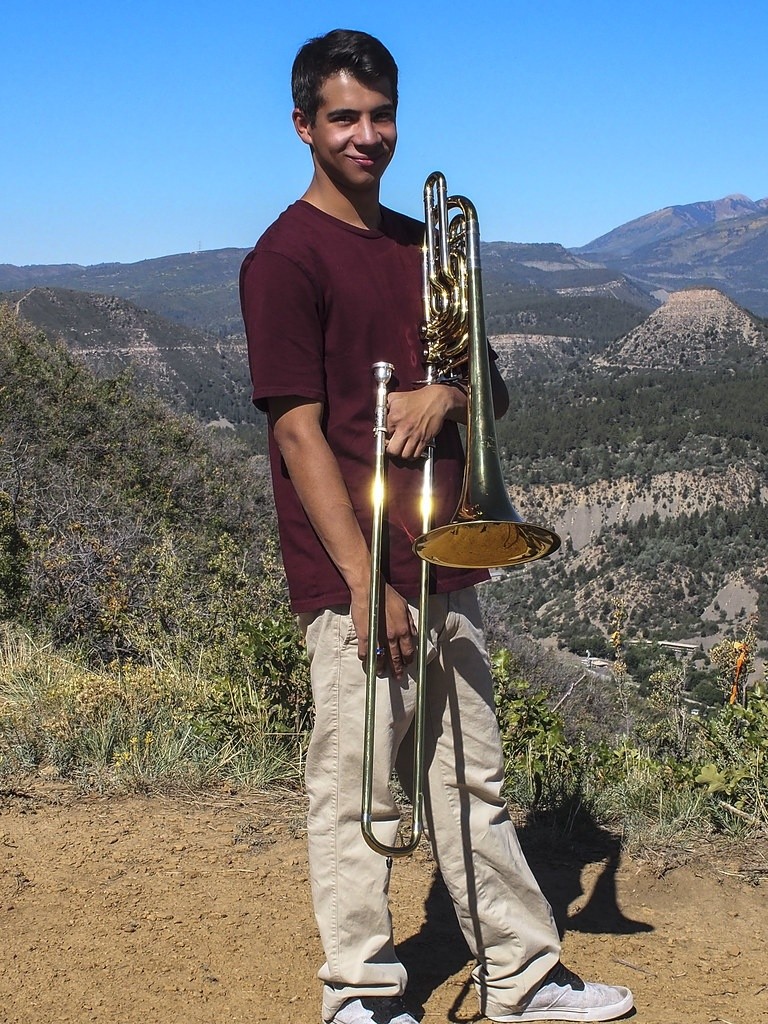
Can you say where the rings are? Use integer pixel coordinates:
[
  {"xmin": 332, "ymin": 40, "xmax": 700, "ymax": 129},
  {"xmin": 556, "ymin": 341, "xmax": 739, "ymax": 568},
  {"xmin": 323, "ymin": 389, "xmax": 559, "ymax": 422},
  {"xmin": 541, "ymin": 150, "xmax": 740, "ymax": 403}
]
[{"xmin": 376, "ymin": 647, "xmax": 387, "ymax": 656}]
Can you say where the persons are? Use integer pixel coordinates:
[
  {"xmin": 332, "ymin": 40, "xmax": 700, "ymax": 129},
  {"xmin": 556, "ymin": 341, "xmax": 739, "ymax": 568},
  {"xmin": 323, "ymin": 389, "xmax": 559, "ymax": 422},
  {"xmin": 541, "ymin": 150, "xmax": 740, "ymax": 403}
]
[{"xmin": 239, "ymin": 30, "xmax": 633, "ymax": 1024}]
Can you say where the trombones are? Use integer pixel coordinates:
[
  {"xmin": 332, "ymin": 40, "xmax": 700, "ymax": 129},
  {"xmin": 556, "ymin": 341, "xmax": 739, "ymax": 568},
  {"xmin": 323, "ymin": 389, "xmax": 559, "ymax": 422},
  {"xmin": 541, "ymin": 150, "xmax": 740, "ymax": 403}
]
[{"xmin": 346, "ymin": 168, "xmax": 573, "ymax": 873}]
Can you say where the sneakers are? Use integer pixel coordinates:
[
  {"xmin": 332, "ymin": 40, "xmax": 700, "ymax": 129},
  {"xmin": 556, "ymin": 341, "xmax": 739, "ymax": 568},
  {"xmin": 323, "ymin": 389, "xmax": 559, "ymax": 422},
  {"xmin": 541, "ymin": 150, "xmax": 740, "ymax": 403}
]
[
  {"xmin": 319, "ymin": 994, "xmax": 417, "ymax": 1024},
  {"xmin": 485, "ymin": 962, "xmax": 635, "ymax": 1023}
]
